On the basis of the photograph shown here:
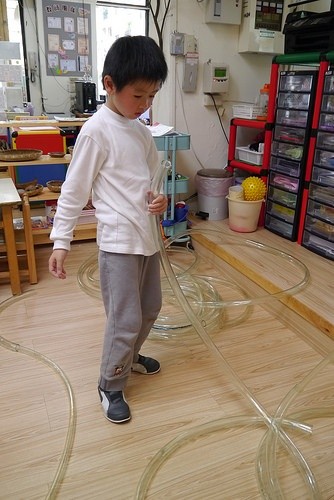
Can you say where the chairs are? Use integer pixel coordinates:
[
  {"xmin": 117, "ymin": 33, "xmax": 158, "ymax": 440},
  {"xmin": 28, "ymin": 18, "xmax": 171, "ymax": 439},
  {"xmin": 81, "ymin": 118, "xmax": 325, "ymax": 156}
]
[{"xmin": 0, "ymin": 189, "xmax": 38, "ymax": 285}]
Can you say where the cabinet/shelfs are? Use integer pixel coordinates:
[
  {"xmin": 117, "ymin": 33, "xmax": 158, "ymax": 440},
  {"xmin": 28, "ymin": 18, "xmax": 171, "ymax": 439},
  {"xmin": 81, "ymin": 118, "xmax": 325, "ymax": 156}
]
[
  {"xmin": 0, "ymin": 154, "xmax": 97, "ymax": 244},
  {"xmin": 227, "ymin": 51, "xmax": 334, "ymax": 261},
  {"xmin": 153, "ymin": 133, "xmax": 194, "ymax": 250}
]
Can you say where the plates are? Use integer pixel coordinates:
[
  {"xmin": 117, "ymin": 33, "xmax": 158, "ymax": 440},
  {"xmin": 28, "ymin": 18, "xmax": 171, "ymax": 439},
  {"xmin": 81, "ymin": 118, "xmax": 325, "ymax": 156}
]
[{"xmin": 48, "ymin": 152, "xmax": 65, "ymax": 158}]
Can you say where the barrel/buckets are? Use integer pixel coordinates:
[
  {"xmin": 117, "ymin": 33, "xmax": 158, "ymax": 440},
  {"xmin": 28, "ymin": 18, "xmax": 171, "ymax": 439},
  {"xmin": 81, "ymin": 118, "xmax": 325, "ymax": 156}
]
[{"xmin": 226, "ymin": 194, "xmax": 266, "ymax": 233}]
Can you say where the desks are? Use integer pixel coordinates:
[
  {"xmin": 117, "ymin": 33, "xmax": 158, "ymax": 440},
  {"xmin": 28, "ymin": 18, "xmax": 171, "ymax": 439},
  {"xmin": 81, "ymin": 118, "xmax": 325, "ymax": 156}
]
[{"xmin": 0, "ymin": 178, "xmax": 22, "ymax": 295}]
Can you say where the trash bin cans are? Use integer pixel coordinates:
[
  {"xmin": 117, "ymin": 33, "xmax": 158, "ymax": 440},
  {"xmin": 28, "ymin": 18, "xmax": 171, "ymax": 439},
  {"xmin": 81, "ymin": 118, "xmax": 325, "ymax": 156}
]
[{"xmin": 194, "ymin": 169, "xmax": 234, "ymax": 221}]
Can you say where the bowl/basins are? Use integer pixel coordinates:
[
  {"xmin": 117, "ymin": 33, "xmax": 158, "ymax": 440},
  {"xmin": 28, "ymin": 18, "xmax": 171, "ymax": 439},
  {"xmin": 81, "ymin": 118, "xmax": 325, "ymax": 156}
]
[
  {"xmin": 46, "ymin": 179, "xmax": 66, "ymax": 192},
  {"xmin": 67, "ymin": 146, "xmax": 74, "ymax": 154}
]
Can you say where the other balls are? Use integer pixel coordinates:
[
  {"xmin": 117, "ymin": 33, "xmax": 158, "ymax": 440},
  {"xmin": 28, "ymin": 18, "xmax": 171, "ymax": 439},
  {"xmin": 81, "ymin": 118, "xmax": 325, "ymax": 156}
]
[{"xmin": 241, "ymin": 176, "xmax": 267, "ymax": 201}]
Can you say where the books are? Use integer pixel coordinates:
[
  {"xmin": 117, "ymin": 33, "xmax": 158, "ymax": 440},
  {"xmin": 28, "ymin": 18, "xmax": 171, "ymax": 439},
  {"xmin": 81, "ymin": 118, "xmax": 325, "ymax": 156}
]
[
  {"xmin": 16, "ymin": 116, "xmax": 48, "ymax": 119},
  {"xmin": 12, "ymin": 216, "xmax": 44, "ymax": 230},
  {"xmin": 45, "ymin": 200, "xmax": 98, "ymax": 225}
]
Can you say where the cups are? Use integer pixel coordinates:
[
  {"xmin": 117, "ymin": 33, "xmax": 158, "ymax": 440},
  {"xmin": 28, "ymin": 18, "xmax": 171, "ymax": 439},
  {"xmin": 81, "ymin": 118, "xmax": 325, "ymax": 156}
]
[{"xmin": 229, "ymin": 186, "xmax": 244, "ymax": 199}]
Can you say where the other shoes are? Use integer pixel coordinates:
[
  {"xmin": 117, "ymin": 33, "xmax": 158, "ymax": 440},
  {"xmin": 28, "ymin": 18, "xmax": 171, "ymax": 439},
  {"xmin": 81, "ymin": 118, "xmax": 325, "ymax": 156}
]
[
  {"xmin": 130, "ymin": 354, "xmax": 161, "ymax": 375},
  {"xmin": 98, "ymin": 386, "xmax": 130, "ymax": 423}
]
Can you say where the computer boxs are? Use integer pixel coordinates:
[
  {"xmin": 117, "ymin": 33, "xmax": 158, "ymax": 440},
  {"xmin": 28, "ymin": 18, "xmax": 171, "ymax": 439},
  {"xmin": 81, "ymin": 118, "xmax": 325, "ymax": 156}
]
[{"xmin": 75, "ymin": 81, "xmax": 96, "ymax": 113}]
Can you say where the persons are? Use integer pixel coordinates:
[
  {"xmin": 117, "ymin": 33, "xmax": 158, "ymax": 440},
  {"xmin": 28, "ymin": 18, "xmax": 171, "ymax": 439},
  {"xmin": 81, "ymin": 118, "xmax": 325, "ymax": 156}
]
[{"xmin": 49, "ymin": 34, "xmax": 167, "ymax": 424}]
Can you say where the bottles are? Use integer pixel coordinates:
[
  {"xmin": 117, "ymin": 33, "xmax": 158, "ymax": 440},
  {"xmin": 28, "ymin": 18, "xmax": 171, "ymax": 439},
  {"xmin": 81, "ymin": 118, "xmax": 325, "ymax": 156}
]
[{"xmin": 257, "ymin": 83, "xmax": 269, "ymax": 120}]
